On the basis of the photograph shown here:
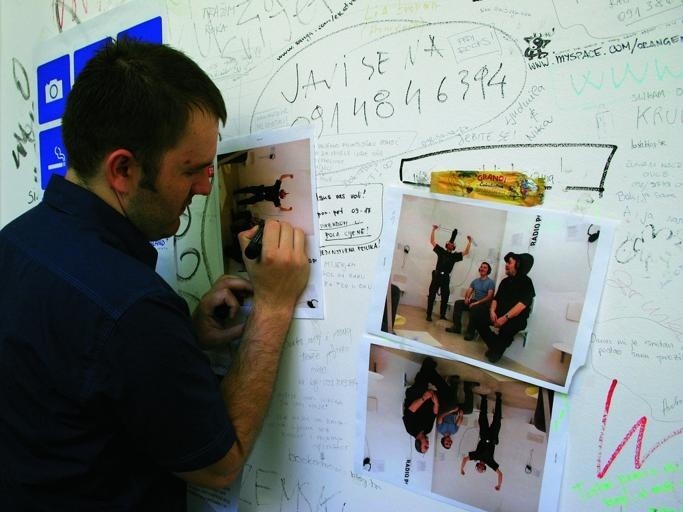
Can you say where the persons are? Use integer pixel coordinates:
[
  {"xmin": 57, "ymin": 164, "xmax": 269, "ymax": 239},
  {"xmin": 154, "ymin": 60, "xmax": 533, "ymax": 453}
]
[
  {"xmin": 0, "ymin": 33, "xmax": 313, "ymax": 510},
  {"xmin": 401, "ymin": 356, "xmax": 456, "ymax": 454},
  {"xmin": 425, "ymin": 224, "xmax": 471, "ymax": 322},
  {"xmin": 229, "ymin": 174, "xmax": 296, "ymax": 212},
  {"xmin": 469, "ymin": 251, "xmax": 535, "ymax": 363},
  {"xmin": 444, "ymin": 262, "xmax": 496, "ymax": 342},
  {"xmin": 436, "ymin": 373, "xmax": 480, "ymax": 449},
  {"xmin": 460, "ymin": 390, "xmax": 503, "ymax": 491}
]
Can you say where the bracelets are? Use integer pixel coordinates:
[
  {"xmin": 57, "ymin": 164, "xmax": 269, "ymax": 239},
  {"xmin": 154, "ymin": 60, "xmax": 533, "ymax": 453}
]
[
  {"xmin": 504, "ymin": 314, "xmax": 510, "ymax": 320},
  {"xmin": 420, "ymin": 396, "xmax": 425, "ymax": 401}
]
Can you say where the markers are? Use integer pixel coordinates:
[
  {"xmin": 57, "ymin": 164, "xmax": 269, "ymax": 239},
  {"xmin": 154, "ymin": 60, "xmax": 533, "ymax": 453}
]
[{"xmin": 245, "ymin": 223, "xmax": 264, "ymax": 259}]
